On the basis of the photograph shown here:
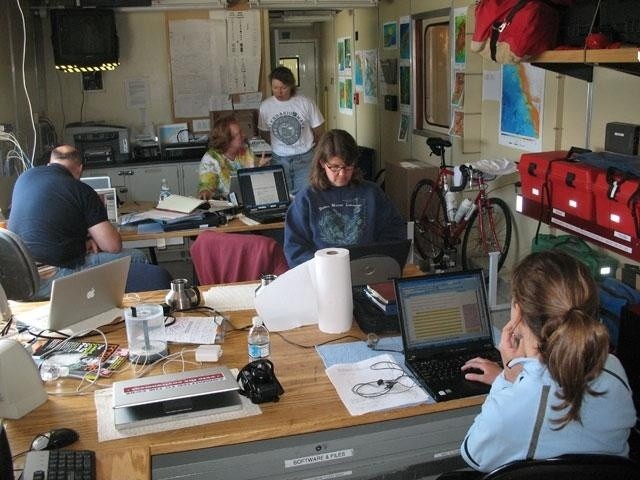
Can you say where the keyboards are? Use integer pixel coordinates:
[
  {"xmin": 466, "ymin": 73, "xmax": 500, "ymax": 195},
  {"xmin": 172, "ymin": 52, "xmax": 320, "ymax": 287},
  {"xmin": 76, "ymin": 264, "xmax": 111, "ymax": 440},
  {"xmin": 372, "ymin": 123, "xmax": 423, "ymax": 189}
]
[{"xmin": 21, "ymin": 449, "xmax": 97, "ymax": 480}]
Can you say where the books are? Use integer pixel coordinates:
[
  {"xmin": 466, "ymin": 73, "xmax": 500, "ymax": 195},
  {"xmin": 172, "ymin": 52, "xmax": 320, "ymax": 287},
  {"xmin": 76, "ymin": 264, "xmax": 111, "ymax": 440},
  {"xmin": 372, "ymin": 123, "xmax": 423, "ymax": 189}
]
[
  {"xmin": 34, "ymin": 339, "xmax": 132, "ymax": 378},
  {"xmin": 363, "ymin": 281, "xmax": 397, "ymax": 315},
  {"xmin": 155, "ymin": 194, "xmax": 233, "ymax": 215}
]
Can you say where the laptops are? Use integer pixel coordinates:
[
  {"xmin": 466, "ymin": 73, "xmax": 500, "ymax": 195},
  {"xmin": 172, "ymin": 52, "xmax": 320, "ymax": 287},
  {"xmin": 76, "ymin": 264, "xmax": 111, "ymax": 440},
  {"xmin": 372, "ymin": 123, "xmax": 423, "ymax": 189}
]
[
  {"xmin": 334, "ymin": 238, "xmax": 412, "ymax": 288},
  {"xmin": 95, "ymin": 188, "xmax": 120, "ymax": 234},
  {"xmin": 65, "ymin": 123, "xmax": 133, "ymax": 167},
  {"xmin": 13, "ymin": 255, "xmax": 132, "ymax": 334},
  {"xmin": 80, "ymin": 176, "xmax": 111, "ymax": 190},
  {"xmin": 392, "ymin": 268, "xmax": 503, "ymax": 403},
  {"xmin": 239, "ymin": 165, "xmax": 292, "ymax": 224},
  {"xmin": 112, "ymin": 365, "xmax": 242, "ymax": 432},
  {"xmin": 229, "ymin": 174, "xmax": 243, "ymax": 207}
]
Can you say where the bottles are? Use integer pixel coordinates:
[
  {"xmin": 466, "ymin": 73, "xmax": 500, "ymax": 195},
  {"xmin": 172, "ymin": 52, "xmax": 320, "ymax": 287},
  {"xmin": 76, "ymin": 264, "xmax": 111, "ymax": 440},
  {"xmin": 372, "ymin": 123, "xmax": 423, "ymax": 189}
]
[
  {"xmin": 455, "ymin": 198, "xmax": 472, "ymax": 222},
  {"xmin": 32, "ymin": 355, "xmax": 70, "ymax": 382},
  {"xmin": 247, "ymin": 317, "xmax": 271, "ymax": 365},
  {"xmin": 159, "ymin": 179, "xmax": 172, "ymax": 199}
]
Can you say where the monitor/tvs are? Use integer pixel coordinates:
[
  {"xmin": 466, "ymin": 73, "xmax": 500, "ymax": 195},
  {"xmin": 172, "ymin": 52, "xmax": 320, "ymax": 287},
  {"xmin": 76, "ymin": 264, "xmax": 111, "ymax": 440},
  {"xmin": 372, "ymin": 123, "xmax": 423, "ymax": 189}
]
[{"xmin": 49, "ymin": 8, "xmax": 120, "ymax": 72}]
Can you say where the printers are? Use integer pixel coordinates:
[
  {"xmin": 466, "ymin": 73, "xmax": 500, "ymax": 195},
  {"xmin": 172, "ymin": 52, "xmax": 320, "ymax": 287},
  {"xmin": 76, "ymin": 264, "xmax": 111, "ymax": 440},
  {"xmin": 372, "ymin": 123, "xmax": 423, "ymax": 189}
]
[{"xmin": 163, "ymin": 140, "xmax": 207, "ymax": 161}]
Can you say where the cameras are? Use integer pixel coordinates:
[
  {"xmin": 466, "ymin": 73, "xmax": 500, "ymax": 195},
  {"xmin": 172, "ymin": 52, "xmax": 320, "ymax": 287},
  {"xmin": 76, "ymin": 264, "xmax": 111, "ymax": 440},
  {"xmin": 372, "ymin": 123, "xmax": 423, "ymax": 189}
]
[{"xmin": 238, "ymin": 358, "xmax": 284, "ymax": 405}]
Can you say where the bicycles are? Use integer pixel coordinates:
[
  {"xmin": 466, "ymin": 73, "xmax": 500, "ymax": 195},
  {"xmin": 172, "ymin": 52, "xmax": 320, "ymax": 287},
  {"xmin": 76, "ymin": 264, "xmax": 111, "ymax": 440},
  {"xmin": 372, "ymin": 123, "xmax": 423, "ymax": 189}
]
[{"xmin": 409, "ymin": 137, "xmax": 512, "ymax": 284}]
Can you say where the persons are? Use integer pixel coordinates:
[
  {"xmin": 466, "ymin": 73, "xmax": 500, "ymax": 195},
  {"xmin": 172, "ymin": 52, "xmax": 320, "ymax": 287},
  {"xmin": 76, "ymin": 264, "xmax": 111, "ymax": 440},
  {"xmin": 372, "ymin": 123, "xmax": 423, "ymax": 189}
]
[
  {"xmin": 282, "ymin": 128, "xmax": 403, "ymax": 271},
  {"xmin": 435, "ymin": 251, "xmax": 637, "ymax": 480},
  {"xmin": 257, "ymin": 66, "xmax": 325, "ymax": 203},
  {"xmin": 8, "ymin": 142, "xmax": 150, "ymax": 303},
  {"xmin": 197, "ymin": 114, "xmax": 273, "ymax": 202}
]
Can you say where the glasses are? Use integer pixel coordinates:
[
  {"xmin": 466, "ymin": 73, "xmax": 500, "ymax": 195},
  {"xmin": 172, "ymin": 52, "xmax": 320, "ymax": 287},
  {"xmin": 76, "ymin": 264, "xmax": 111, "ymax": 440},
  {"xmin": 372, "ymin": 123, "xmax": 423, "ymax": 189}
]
[{"xmin": 324, "ymin": 160, "xmax": 355, "ymax": 172}]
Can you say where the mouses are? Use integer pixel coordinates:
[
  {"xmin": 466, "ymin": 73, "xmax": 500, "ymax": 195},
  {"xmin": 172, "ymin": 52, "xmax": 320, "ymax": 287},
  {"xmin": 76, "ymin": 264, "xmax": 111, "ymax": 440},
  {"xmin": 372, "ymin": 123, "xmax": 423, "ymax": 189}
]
[{"xmin": 32, "ymin": 428, "xmax": 79, "ymax": 451}]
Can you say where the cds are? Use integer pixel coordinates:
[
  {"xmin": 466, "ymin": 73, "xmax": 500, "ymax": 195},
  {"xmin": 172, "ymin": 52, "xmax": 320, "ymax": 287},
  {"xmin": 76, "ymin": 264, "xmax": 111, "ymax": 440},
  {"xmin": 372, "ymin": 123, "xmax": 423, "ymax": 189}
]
[{"xmin": 128, "ymin": 340, "xmax": 167, "ymax": 365}]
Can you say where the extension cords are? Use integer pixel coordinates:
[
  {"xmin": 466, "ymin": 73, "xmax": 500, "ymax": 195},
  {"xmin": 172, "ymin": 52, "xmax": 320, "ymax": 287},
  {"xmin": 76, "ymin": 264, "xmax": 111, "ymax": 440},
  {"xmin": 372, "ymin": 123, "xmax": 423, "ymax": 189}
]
[{"xmin": 37, "ymin": 122, "xmax": 51, "ymax": 158}]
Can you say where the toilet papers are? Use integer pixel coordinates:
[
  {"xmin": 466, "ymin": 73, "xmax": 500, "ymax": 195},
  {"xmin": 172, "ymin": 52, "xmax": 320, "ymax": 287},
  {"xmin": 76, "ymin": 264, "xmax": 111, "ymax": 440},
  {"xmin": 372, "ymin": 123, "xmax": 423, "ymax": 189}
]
[{"xmin": 256, "ymin": 249, "xmax": 356, "ymax": 334}]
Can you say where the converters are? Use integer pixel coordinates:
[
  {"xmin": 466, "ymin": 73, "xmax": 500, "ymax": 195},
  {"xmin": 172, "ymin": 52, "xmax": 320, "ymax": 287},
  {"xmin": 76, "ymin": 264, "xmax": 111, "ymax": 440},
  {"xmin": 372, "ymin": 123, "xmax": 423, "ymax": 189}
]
[{"xmin": 195, "ymin": 344, "xmax": 222, "ymax": 363}]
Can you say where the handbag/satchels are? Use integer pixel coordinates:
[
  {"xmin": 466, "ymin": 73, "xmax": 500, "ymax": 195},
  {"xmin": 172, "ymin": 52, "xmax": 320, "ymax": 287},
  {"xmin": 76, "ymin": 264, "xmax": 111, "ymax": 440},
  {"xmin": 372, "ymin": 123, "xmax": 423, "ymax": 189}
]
[{"xmin": 471, "ymin": 0, "xmax": 559, "ymax": 65}]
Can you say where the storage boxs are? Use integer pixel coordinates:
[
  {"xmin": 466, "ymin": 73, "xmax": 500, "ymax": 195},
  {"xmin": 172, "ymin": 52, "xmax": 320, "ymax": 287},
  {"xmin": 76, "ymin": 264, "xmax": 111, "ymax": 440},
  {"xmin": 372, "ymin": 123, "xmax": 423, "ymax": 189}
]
[
  {"xmin": 592, "ymin": 172, "xmax": 640, "ymax": 239},
  {"xmin": 384, "ymin": 160, "xmax": 439, "ymax": 225},
  {"xmin": 516, "ymin": 149, "xmax": 566, "ymax": 206},
  {"xmin": 547, "ymin": 160, "xmax": 599, "ymax": 222}
]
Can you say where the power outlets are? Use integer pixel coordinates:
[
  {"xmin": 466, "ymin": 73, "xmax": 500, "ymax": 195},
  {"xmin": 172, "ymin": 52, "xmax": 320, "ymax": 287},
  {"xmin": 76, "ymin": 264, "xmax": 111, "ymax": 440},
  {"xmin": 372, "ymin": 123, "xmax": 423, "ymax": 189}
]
[{"xmin": 33, "ymin": 113, "xmax": 43, "ymax": 125}]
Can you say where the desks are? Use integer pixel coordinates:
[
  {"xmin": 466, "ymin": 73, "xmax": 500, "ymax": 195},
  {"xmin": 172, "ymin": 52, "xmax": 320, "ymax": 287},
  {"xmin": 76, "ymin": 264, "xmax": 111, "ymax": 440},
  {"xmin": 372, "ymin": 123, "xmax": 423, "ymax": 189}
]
[
  {"xmin": 117, "ymin": 199, "xmax": 285, "ymax": 241},
  {"xmin": 1, "ymin": 264, "xmax": 492, "ymax": 480}
]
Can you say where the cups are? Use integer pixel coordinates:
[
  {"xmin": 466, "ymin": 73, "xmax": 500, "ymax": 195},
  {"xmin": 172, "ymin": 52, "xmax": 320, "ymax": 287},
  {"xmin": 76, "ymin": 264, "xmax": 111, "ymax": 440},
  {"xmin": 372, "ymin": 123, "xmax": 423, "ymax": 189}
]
[{"xmin": 125, "ymin": 304, "xmax": 167, "ymax": 364}]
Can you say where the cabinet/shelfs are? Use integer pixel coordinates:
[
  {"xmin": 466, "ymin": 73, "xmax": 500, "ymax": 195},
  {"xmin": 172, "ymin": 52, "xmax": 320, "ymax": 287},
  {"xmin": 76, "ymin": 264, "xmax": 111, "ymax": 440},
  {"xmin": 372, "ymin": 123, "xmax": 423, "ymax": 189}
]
[{"xmin": 84, "ymin": 159, "xmax": 203, "ymax": 202}]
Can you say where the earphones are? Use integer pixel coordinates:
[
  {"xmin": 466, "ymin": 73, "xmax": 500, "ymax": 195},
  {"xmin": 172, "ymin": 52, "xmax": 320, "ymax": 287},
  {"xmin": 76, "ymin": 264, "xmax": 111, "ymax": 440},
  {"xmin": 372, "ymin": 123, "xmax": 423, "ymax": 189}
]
[{"xmin": 377, "ymin": 379, "xmax": 384, "ymax": 387}]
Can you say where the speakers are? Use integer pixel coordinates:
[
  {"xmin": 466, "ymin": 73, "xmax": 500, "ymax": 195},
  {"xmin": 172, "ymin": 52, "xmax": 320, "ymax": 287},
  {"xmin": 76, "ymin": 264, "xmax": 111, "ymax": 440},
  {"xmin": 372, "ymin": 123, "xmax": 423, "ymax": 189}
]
[
  {"xmin": 604, "ymin": 122, "xmax": 640, "ymax": 156},
  {"xmin": 0, "ymin": 338, "xmax": 48, "ymax": 420}
]
